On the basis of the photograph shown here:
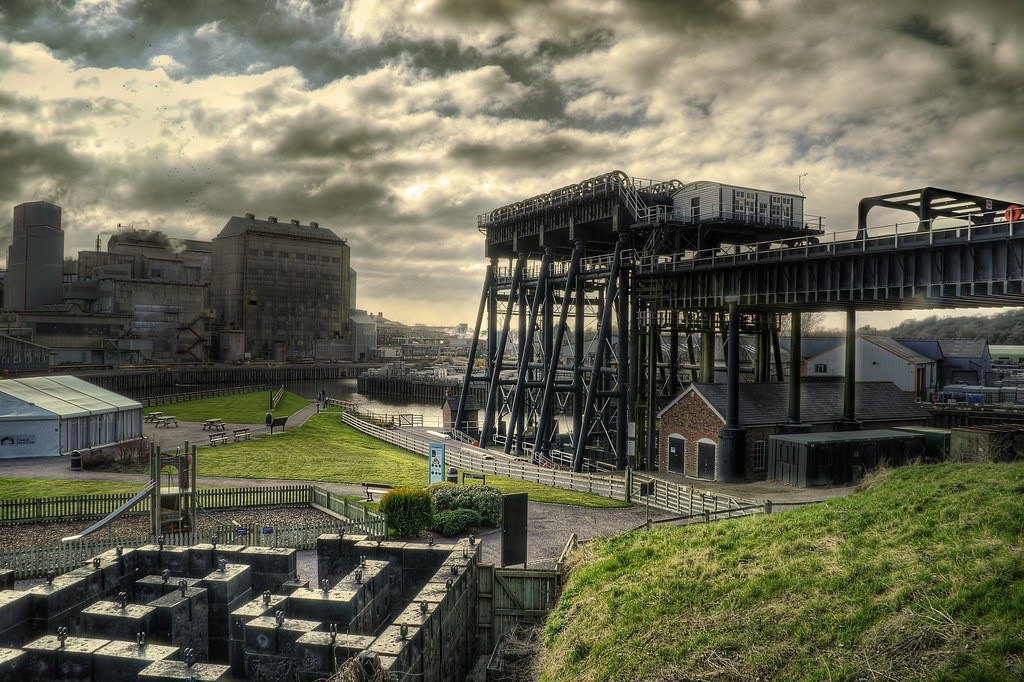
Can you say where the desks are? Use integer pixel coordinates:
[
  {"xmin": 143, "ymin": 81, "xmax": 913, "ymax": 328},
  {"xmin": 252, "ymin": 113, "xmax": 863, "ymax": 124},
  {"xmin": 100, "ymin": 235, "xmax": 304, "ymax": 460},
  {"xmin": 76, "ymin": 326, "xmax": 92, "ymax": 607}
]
[
  {"xmin": 148, "ymin": 412, "xmax": 163, "ymax": 423},
  {"xmin": 153, "ymin": 416, "xmax": 180, "ymax": 429},
  {"xmin": 202, "ymin": 419, "xmax": 226, "ymax": 431}
]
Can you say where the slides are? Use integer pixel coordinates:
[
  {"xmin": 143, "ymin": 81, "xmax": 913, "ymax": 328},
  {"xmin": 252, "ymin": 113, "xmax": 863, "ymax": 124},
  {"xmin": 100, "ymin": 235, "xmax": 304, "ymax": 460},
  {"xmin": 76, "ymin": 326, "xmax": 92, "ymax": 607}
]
[{"xmin": 59, "ymin": 480, "xmax": 156, "ymax": 544}]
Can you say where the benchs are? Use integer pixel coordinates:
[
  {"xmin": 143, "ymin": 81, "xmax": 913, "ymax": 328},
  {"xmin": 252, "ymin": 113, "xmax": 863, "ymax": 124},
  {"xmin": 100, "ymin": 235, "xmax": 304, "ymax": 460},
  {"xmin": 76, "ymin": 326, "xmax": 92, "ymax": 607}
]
[
  {"xmin": 232, "ymin": 428, "xmax": 251, "ymax": 442},
  {"xmin": 201, "ymin": 423, "xmax": 227, "ymax": 426},
  {"xmin": 361, "ymin": 482, "xmax": 402, "ymax": 502},
  {"xmin": 144, "ymin": 417, "xmax": 180, "ymax": 429},
  {"xmin": 209, "ymin": 432, "xmax": 229, "ymax": 446}
]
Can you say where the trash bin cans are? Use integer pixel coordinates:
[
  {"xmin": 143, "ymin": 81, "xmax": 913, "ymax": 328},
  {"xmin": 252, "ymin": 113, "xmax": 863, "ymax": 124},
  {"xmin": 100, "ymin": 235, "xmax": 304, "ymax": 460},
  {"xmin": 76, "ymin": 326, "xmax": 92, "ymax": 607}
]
[
  {"xmin": 266, "ymin": 413, "xmax": 272, "ymax": 426},
  {"xmin": 447, "ymin": 468, "xmax": 459, "ymax": 482},
  {"xmin": 70, "ymin": 451, "xmax": 83, "ymax": 471}
]
